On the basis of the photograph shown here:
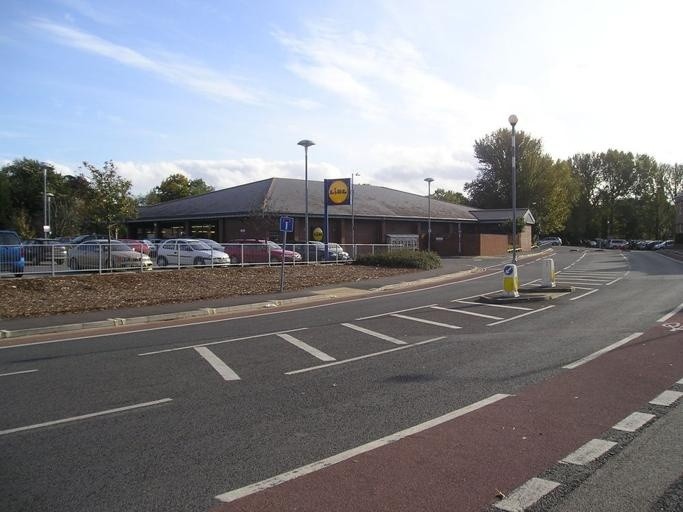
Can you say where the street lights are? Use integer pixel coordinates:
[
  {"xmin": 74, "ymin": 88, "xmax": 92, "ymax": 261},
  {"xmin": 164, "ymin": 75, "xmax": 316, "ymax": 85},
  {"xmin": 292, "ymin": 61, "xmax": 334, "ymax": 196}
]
[
  {"xmin": 508, "ymin": 114, "xmax": 519, "ymax": 263},
  {"xmin": 40, "ymin": 164, "xmax": 54, "ymax": 238},
  {"xmin": 298, "ymin": 140, "xmax": 315, "ymax": 261},
  {"xmin": 352, "ymin": 171, "xmax": 360, "ymax": 259},
  {"xmin": 424, "ymin": 178, "xmax": 435, "ymax": 252}
]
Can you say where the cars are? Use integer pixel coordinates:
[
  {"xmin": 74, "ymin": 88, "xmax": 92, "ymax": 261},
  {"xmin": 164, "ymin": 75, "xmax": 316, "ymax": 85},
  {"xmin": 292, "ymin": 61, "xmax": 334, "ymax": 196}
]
[
  {"xmin": 586, "ymin": 238, "xmax": 674, "ymax": 250},
  {"xmin": 539, "ymin": 237, "xmax": 562, "ymax": 246}
]
